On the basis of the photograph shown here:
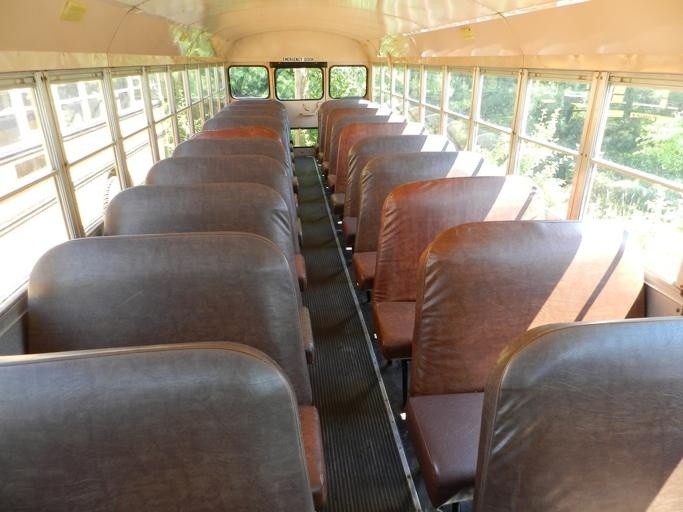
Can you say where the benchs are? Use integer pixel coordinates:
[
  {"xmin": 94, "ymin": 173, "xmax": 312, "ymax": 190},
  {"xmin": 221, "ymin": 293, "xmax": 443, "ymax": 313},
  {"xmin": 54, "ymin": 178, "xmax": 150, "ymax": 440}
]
[{"xmin": 0, "ymin": 98, "xmax": 683, "ymax": 512}]
